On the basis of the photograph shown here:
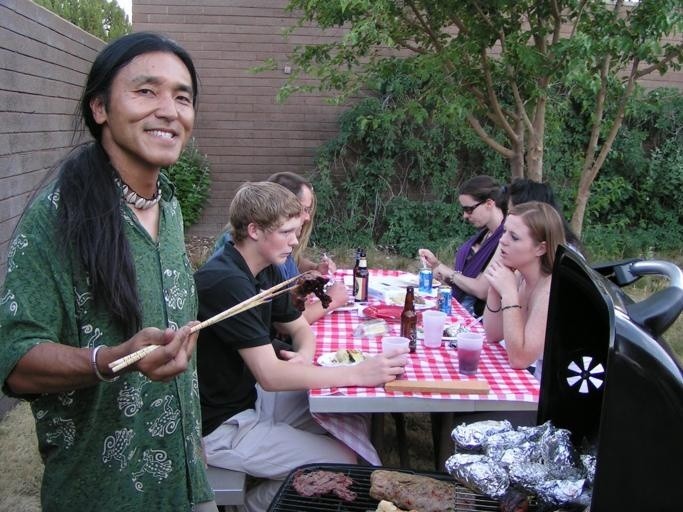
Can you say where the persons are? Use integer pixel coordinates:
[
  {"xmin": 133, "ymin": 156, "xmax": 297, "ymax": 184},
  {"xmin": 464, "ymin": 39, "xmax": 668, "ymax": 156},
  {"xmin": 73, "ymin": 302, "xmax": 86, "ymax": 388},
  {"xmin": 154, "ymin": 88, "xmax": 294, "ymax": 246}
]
[
  {"xmin": 503, "ymin": 178, "xmax": 586, "ymax": 292},
  {"xmin": 0, "ymin": 33, "xmax": 224, "ymax": 512},
  {"xmin": 418, "ymin": 176, "xmax": 506, "ymax": 321},
  {"xmin": 202, "ymin": 172, "xmax": 349, "ymax": 328},
  {"xmin": 483, "ymin": 201, "xmax": 586, "ymax": 387},
  {"xmin": 192, "ymin": 179, "xmax": 411, "ymax": 511}
]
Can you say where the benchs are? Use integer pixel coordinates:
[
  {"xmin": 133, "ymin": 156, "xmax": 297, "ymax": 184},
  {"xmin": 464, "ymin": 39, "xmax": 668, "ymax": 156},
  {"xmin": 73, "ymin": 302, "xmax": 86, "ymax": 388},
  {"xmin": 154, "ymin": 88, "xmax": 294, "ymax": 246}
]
[{"xmin": 205, "ymin": 461, "xmax": 247, "ymax": 506}]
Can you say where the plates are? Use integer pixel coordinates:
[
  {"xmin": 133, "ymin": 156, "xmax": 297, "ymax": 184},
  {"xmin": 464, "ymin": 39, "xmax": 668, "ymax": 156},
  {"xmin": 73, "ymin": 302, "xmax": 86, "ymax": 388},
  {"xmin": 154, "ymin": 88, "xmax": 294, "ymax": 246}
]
[
  {"xmin": 363, "ymin": 304, "xmax": 423, "ymax": 324},
  {"xmin": 371, "ymin": 273, "xmax": 441, "ymax": 309},
  {"xmin": 316, "ymin": 352, "xmax": 374, "ymax": 367},
  {"xmin": 417, "ymin": 324, "xmax": 459, "ymax": 341},
  {"xmin": 312, "ymin": 289, "xmax": 360, "ymax": 311}
]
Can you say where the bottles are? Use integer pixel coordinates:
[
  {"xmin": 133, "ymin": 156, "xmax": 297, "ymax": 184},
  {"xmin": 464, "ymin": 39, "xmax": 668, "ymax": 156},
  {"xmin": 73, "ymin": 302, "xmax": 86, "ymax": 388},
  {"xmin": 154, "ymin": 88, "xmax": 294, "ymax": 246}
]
[
  {"xmin": 401, "ymin": 285, "xmax": 417, "ymax": 354},
  {"xmin": 353, "ymin": 248, "xmax": 368, "ymax": 301}
]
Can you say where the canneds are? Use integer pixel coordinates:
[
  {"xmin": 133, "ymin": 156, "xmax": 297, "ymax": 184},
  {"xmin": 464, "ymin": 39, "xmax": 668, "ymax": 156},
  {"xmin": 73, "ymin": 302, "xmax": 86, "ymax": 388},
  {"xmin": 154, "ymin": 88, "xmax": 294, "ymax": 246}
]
[
  {"xmin": 437, "ymin": 285, "xmax": 453, "ymax": 316},
  {"xmin": 419, "ymin": 268, "xmax": 432, "ymax": 294}
]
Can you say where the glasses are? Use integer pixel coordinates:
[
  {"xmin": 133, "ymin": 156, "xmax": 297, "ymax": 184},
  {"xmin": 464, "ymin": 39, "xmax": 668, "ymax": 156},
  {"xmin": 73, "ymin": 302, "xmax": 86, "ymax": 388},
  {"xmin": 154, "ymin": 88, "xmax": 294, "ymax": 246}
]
[{"xmin": 461, "ymin": 197, "xmax": 495, "ymax": 214}]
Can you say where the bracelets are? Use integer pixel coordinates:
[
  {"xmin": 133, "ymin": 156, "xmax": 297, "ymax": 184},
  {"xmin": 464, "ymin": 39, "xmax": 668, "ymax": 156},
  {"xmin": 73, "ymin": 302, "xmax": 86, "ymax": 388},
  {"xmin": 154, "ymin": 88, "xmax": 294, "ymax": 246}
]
[
  {"xmin": 450, "ymin": 271, "xmax": 463, "ymax": 285},
  {"xmin": 501, "ymin": 304, "xmax": 520, "ymax": 311},
  {"xmin": 486, "ymin": 303, "xmax": 501, "ymax": 313},
  {"xmin": 91, "ymin": 344, "xmax": 120, "ymax": 383}
]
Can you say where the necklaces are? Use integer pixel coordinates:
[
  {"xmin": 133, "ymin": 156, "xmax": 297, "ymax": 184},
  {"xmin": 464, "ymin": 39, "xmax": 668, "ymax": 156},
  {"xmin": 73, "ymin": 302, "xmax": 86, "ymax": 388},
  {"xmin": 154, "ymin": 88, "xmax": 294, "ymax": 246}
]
[{"xmin": 106, "ymin": 158, "xmax": 162, "ymax": 210}]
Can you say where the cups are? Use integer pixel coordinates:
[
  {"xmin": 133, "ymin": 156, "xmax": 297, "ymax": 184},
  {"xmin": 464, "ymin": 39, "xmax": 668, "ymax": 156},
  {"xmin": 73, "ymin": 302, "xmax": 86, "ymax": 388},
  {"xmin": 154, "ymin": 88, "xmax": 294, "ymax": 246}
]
[
  {"xmin": 382, "ymin": 336, "xmax": 410, "ymax": 378},
  {"xmin": 422, "ymin": 309, "xmax": 447, "ymax": 348},
  {"xmin": 457, "ymin": 332, "xmax": 483, "ymax": 375}
]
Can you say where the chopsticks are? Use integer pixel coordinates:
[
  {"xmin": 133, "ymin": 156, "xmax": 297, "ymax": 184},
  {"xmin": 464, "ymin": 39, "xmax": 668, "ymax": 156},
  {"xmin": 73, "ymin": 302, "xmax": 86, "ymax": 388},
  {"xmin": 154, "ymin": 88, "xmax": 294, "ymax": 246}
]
[{"xmin": 107, "ymin": 271, "xmax": 305, "ymax": 372}]
